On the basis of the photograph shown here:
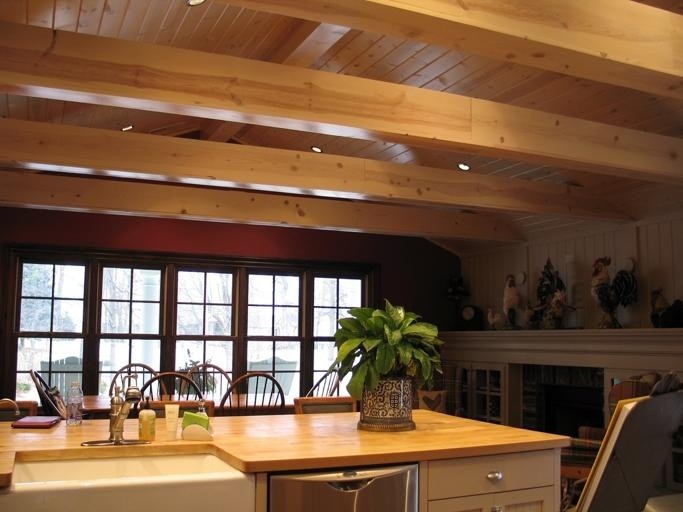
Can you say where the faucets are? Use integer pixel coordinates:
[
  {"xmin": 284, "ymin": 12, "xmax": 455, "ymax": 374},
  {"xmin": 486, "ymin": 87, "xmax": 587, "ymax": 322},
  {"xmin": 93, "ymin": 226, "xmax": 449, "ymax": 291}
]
[{"xmin": 108, "ymin": 385, "xmax": 141, "ymax": 441}]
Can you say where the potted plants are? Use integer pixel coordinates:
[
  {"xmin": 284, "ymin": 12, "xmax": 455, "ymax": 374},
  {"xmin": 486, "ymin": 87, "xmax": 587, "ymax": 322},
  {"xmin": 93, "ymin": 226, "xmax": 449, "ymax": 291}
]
[{"xmin": 326, "ymin": 301, "xmax": 441, "ymax": 432}]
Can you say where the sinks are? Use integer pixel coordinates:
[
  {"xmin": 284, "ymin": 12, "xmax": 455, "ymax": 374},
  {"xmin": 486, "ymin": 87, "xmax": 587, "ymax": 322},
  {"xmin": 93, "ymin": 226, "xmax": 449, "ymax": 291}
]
[{"xmin": 9, "ymin": 453, "xmax": 256, "ymax": 512}]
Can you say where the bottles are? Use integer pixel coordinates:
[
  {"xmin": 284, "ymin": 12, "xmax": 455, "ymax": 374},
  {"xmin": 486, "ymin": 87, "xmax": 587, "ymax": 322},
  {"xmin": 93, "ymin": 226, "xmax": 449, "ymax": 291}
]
[
  {"xmin": 196, "ymin": 398, "xmax": 208, "ymax": 416},
  {"xmin": 65, "ymin": 381, "xmax": 83, "ymax": 427},
  {"xmin": 137, "ymin": 396, "xmax": 157, "ymax": 442}
]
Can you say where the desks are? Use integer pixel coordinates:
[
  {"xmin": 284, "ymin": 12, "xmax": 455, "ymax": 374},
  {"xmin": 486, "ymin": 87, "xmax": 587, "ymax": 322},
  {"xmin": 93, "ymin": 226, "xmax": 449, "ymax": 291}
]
[
  {"xmin": 0, "ymin": 409, "xmax": 571, "ymax": 512},
  {"xmin": 59, "ymin": 394, "xmax": 296, "ymax": 417}
]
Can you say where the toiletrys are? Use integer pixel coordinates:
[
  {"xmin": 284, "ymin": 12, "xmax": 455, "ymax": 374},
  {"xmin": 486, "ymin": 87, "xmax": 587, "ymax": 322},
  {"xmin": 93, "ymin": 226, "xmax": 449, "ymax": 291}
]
[
  {"xmin": 164, "ymin": 403, "xmax": 180, "ymax": 441},
  {"xmin": 138, "ymin": 396, "xmax": 155, "ymax": 442}
]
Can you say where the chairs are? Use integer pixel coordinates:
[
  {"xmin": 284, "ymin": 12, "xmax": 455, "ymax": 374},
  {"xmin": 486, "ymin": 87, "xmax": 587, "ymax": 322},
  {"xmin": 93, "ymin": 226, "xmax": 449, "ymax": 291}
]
[
  {"xmin": 135, "ymin": 372, "xmax": 203, "ymax": 409},
  {"xmin": 110, "ymin": 362, "xmax": 168, "ymax": 397},
  {"xmin": 303, "ymin": 368, "xmax": 343, "ymax": 398},
  {"xmin": 139, "ymin": 399, "xmax": 217, "ymax": 416},
  {"xmin": 181, "ymin": 365, "xmax": 240, "ymax": 397},
  {"xmin": 15, "ymin": 401, "xmax": 38, "ymax": 417},
  {"xmin": 216, "ymin": 373, "xmax": 285, "ymax": 417},
  {"xmin": 30, "ymin": 369, "xmax": 67, "ymax": 420},
  {"xmin": 294, "ymin": 398, "xmax": 360, "ymax": 413}
]
